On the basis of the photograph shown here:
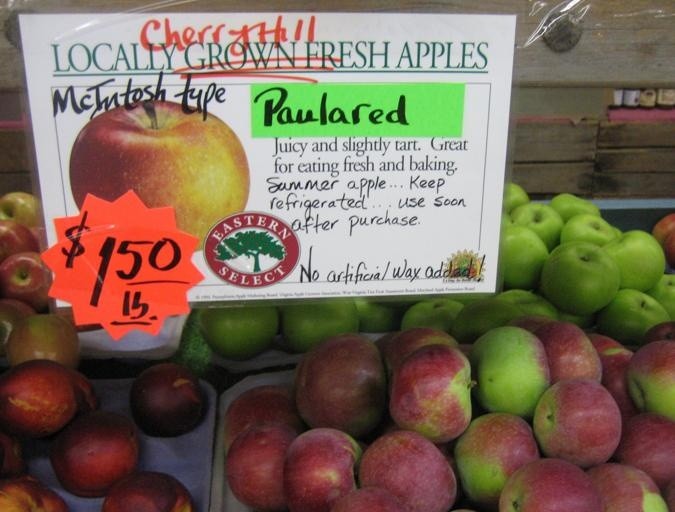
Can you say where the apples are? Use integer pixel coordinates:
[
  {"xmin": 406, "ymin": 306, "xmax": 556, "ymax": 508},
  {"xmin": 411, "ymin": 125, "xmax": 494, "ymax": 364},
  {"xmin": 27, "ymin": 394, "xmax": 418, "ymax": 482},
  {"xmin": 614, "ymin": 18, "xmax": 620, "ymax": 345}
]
[
  {"xmin": 1, "ymin": 180, "xmax": 674, "ymax": 512},
  {"xmin": 68, "ymin": 100, "xmax": 250, "ymax": 252}
]
